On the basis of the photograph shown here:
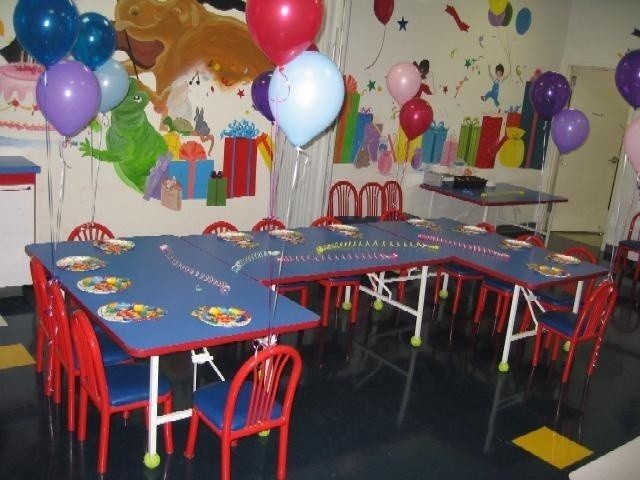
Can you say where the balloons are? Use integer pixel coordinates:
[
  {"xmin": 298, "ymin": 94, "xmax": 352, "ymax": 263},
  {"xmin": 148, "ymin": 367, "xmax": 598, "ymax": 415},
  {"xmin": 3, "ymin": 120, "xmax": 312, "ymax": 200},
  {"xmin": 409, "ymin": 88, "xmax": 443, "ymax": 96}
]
[
  {"xmin": 385, "ymin": 62, "xmax": 434, "ymax": 209},
  {"xmin": 599, "ymin": 48, "xmax": 640, "ymax": 287},
  {"xmin": 254, "ymin": 1, "xmax": 346, "ymax": 394},
  {"xmin": 488, "ymin": 0, "xmax": 534, "ymax": 77},
  {"xmin": 529, "ymin": 70, "xmax": 590, "ymax": 238},
  {"xmin": 363, "ymin": 0, "xmax": 394, "ymax": 72},
  {"xmin": 12, "ymin": 0, "xmax": 131, "ymax": 318}
]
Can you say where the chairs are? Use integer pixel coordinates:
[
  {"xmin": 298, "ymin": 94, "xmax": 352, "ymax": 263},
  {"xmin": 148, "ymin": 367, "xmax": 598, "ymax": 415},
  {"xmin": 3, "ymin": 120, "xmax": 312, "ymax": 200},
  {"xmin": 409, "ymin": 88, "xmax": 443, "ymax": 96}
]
[{"xmin": 617, "ymin": 211, "xmax": 640, "ymax": 281}]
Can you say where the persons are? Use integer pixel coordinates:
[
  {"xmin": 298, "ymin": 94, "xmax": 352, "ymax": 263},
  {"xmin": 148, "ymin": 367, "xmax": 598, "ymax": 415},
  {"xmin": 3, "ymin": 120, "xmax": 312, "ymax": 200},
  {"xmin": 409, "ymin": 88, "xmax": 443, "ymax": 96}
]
[{"xmin": 481, "ymin": 64, "xmax": 510, "ymax": 112}]
